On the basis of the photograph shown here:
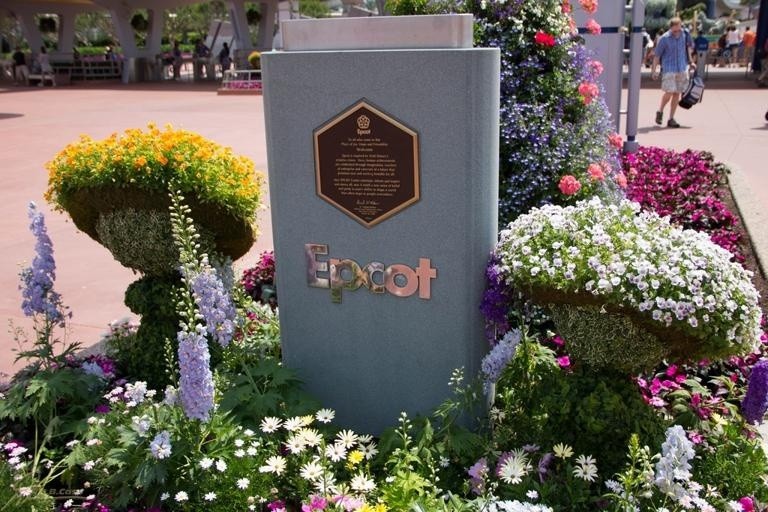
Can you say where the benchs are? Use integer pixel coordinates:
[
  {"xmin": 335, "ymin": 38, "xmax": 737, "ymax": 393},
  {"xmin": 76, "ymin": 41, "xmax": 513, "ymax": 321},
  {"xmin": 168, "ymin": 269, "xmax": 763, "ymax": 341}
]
[{"xmin": 27, "ymin": 68, "xmax": 71, "ymax": 86}]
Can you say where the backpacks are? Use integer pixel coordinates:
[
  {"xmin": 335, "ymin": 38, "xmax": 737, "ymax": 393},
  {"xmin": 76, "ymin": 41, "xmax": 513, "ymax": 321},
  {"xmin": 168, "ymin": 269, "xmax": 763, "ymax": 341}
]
[
  {"xmin": 679, "ymin": 73, "xmax": 705, "ymax": 109},
  {"xmin": 718, "ymin": 34, "xmax": 727, "ymax": 47}
]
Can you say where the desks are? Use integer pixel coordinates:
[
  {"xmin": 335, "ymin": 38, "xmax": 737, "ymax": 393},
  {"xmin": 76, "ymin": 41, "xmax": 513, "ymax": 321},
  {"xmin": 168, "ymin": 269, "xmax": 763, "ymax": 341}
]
[{"xmin": 183, "ymin": 57, "xmax": 220, "ymax": 80}]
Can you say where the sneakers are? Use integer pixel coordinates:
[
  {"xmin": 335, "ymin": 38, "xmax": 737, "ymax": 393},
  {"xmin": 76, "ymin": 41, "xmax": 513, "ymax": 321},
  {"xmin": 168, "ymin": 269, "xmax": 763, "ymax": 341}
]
[
  {"xmin": 668, "ymin": 119, "xmax": 679, "ymax": 127},
  {"xmin": 655, "ymin": 111, "xmax": 663, "ymax": 123}
]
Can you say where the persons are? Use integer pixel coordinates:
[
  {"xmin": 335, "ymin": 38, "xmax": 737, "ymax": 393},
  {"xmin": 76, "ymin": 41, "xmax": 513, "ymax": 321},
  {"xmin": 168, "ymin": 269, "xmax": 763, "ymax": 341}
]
[
  {"xmin": 651, "ymin": 18, "xmax": 703, "ymax": 128},
  {"xmin": 73, "ymin": 48, "xmax": 80, "ymax": 59},
  {"xmin": 194, "ymin": 39, "xmax": 210, "ymax": 77},
  {"xmin": 620, "ymin": 25, "xmax": 665, "ymax": 70},
  {"xmin": 219, "ymin": 42, "xmax": 231, "ymax": 77},
  {"xmin": 173, "ymin": 41, "xmax": 184, "ymax": 80},
  {"xmin": 718, "ymin": 24, "xmax": 757, "ymax": 66},
  {"xmin": 12, "ymin": 47, "xmax": 30, "ymax": 85},
  {"xmin": 755, "ymin": 40, "xmax": 768, "ymax": 88},
  {"xmin": 36, "ymin": 47, "xmax": 56, "ymax": 87},
  {"xmin": 695, "ymin": 31, "xmax": 707, "ymax": 72}
]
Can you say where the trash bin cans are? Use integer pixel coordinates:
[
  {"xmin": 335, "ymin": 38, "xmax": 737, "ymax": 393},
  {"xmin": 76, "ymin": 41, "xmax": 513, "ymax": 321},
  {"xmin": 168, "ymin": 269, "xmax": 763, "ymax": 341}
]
[{"xmin": 123, "ymin": 56, "xmax": 145, "ymax": 84}]
